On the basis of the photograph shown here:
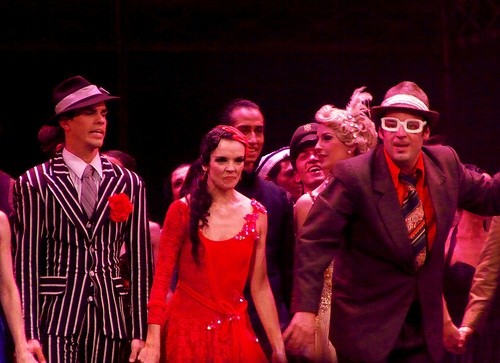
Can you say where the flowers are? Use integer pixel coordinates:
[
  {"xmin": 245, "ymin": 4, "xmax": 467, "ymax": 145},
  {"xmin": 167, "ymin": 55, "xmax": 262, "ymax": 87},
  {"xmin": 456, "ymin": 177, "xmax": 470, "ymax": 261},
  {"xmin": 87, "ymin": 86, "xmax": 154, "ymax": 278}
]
[{"xmin": 105, "ymin": 191, "xmax": 136, "ymax": 225}]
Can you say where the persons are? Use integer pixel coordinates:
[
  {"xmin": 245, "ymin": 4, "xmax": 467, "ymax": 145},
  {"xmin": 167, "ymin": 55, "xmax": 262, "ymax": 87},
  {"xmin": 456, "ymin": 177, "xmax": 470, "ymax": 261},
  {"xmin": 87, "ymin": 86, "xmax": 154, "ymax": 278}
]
[
  {"xmin": 283, "ymin": 80, "xmax": 500, "ymax": 363},
  {"xmin": 103, "ymin": 151, "xmax": 161, "ymax": 295},
  {"xmin": 138, "ymin": 125, "xmax": 287, "ymax": 363},
  {"xmin": 169, "ymin": 99, "xmax": 304, "ymax": 363},
  {"xmin": 9, "ymin": 76, "xmax": 152, "ymax": 363},
  {"xmin": 0, "ymin": 211, "xmax": 37, "ymax": 363}
]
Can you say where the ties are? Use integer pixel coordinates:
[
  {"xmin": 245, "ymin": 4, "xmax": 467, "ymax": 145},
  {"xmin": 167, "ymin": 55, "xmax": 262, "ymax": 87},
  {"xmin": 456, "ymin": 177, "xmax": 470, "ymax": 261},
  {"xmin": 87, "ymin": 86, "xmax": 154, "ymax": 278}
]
[
  {"xmin": 81, "ymin": 164, "xmax": 99, "ymax": 219},
  {"xmin": 397, "ymin": 172, "xmax": 427, "ymax": 268}
]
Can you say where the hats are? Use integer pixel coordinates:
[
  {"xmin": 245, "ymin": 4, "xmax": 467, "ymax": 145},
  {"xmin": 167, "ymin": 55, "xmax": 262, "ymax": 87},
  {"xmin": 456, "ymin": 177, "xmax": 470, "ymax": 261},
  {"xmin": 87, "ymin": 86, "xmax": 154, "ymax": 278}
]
[
  {"xmin": 52, "ymin": 75, "xmax": 122, "ymax": 119},
  {"xmin": 372, "ymin": 81, "xmax": 438, "ymax": 133},
  {"xmin": 255, "ymin": 147, "xmax": 290, "ymax": 180},
  {"xmin": 289, "ymin": 123, "xmax": 320, "ymax": 170}
]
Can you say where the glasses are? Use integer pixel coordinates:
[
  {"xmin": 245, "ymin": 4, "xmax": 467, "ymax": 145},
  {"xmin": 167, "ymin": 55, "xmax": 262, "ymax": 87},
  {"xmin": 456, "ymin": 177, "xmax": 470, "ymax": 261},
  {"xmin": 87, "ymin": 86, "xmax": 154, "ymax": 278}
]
[{"xmin": 380, "ymin": 117, "xmax": 427, "ymax": 133}]
